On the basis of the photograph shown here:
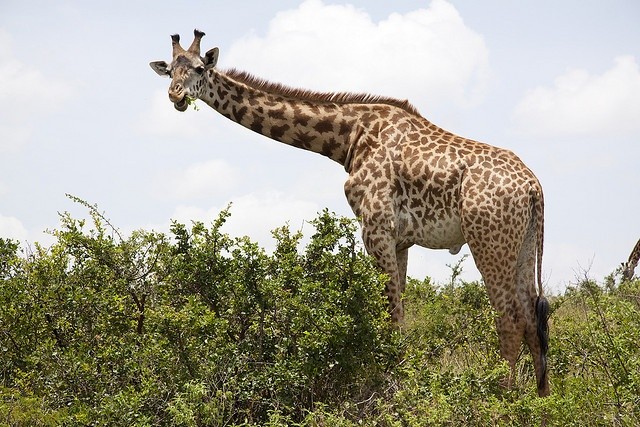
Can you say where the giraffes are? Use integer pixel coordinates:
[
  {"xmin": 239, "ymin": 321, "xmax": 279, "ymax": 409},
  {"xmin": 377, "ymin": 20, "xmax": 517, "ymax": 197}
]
[{"xmin": 149, "ymin": 28, "xmax": 552, "ymax": 397}]
